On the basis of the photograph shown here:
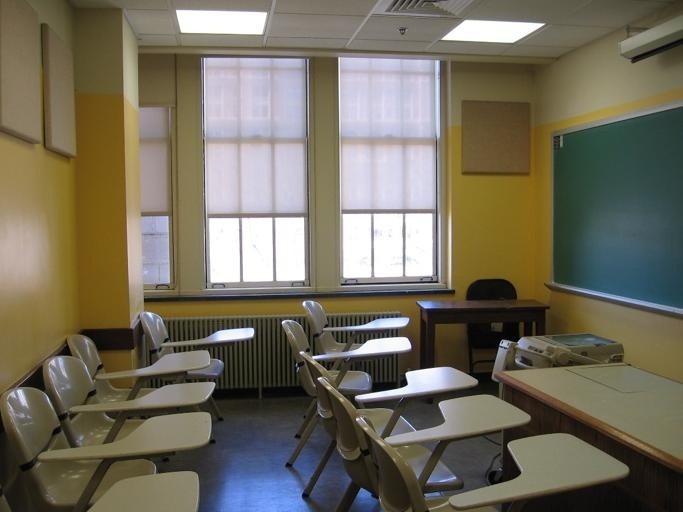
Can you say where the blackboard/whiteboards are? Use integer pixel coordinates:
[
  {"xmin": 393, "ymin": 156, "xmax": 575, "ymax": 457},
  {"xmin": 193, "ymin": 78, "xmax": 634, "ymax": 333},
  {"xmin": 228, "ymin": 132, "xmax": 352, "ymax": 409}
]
[{"xmin": 550, "ymin": 99, "xmax": 683, "ymax": 314}]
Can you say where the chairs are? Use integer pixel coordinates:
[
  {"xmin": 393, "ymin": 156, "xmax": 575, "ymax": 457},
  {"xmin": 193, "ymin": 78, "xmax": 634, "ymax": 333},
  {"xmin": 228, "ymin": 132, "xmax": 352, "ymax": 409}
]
[
  {"xmin": 43, "ymin": 356, "xmax": 147, "ymax": 448},
  {"xmin": 355, "ymin": 417, "xmax": 498, "ymax": 512},
  {"xmin": 1, "ymin": 387, "xmax": 156, "ymax": 506},
  {"xmin": 303, "ymin": 300, "xmax": 364, "ymax": 419},
  {"xmin": 67, "ymin": 334, "xmax": 175, "ymax": 461},
  {"xmin": 318, "ymin": 377, "xmax": 464, "ymax": 512},
  {"xmin": 140, "ymin": 312, "xmax": 224, "ymax": 445},
  {"xmin": 0, "ymin": 484, "xmax": 14, "ymax": 512},
  {"xmin": 300, "ymin": 352, "xmax": 417, "ymax": 500},
  {"xmin": 466, "ymin": 279, "xmax": 520, "ymax": 376},
  {"xmin": 283, "ymin": 321, "xmax": 373, "ymax": 468}
]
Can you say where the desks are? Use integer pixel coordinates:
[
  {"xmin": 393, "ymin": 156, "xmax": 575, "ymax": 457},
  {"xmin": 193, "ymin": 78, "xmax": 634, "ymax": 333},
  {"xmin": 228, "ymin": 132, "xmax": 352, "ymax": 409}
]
[
  {"xmin": 416, "ymin": 300, "xmax": 552, "ymax": 405},
  {"xmin": 494, "ymin": 363, "xmax": 683, "ymax": 512}
]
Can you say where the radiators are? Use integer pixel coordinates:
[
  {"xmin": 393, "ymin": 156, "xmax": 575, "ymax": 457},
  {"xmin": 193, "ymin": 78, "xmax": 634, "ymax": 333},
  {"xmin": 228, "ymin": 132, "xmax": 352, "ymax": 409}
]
[{"xmin": 148, "ymin": 313, "xmax": 400, "ymax": 399}]
